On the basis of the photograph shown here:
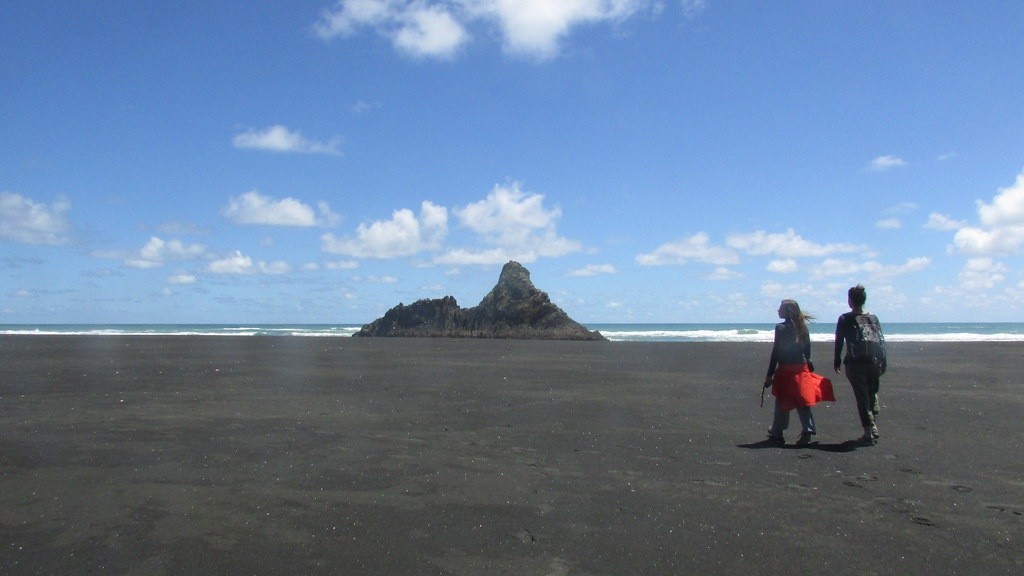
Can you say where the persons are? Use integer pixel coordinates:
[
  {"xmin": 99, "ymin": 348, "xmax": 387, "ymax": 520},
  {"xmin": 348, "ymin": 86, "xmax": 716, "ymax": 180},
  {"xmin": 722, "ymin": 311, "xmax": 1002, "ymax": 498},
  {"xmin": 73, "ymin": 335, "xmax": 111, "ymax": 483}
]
[
  {"xmin": 764, "ymin": 301, "xmax": 816, "ymax": 446},
  {"xmin": 834, "ymin": 284, "xmax": 887, "ymax": 446}
]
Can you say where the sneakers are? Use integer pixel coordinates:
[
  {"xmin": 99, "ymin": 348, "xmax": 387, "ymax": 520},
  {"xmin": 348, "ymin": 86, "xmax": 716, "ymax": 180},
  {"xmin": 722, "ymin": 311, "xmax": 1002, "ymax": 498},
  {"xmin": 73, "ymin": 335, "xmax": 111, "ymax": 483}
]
[
  {"xmin": 768, "ymin": 425, "xmax": 784, "ymax": 440},
  {"xmin": 871, "ymin": 433, "xmax": 879, "ymax": 438},
  {"xmin": 796, "ymin": 433, "xmax": 811, "ymax": 446},
  {"xmin": 858, "ymin": 435, "xmax": 876, "ymax": 445}
]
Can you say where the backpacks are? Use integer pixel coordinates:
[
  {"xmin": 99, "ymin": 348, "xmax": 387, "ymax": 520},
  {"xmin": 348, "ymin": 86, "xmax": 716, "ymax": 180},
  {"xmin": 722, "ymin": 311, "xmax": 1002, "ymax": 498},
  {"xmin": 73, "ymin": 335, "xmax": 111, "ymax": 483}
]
[{"xmin": 843, "ymin": 311, "xmax": 886, "ymax": 379}]
